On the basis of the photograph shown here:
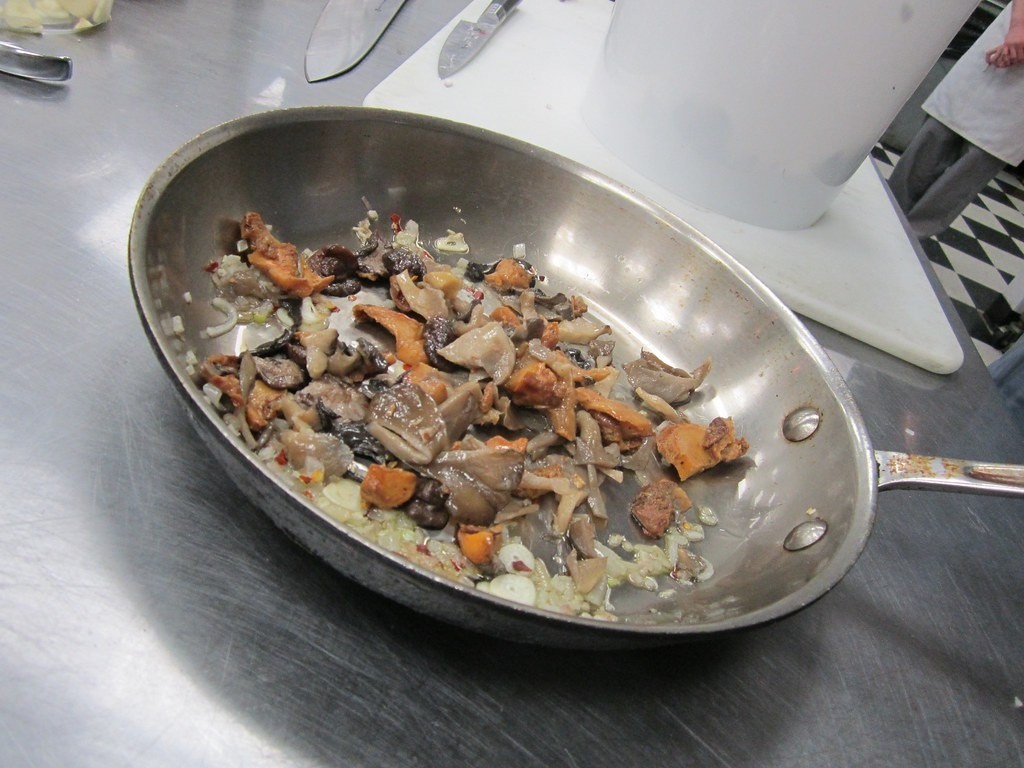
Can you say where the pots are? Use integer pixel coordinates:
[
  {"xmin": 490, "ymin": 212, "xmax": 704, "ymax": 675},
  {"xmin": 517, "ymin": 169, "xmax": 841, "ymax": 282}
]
[{"xmin": 130, "ymin": 107, "xmax": 1024, "ymax": 635}]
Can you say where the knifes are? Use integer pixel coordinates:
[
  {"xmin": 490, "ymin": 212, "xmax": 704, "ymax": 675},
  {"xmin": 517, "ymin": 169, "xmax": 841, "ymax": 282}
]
[
  {"xmin": 305, "ymin": 0, "xmax": 406, "ymax": 82},
  {"xmin": 438, "ymin": 0, "xmax": 525, "ymax": 81}
]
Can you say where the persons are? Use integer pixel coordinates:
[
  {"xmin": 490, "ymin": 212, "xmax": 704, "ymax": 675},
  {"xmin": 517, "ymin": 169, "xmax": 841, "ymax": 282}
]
[{"xmin": 888, "ymin": 0, "xmax": 1024, "ymax": 250}]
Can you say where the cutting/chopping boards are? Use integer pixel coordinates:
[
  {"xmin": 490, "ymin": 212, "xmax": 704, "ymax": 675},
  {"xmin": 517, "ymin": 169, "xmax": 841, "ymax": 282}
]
[{"xmin": 361, "ymin": 1, "xmax": 965, "ymax": 376}]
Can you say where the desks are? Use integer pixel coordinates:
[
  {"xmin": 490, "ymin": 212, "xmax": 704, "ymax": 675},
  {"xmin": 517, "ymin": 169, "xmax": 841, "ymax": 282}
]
[{"xmin": 0, "ymin": 1, "xmax": 1024, "ymax": 768}]
